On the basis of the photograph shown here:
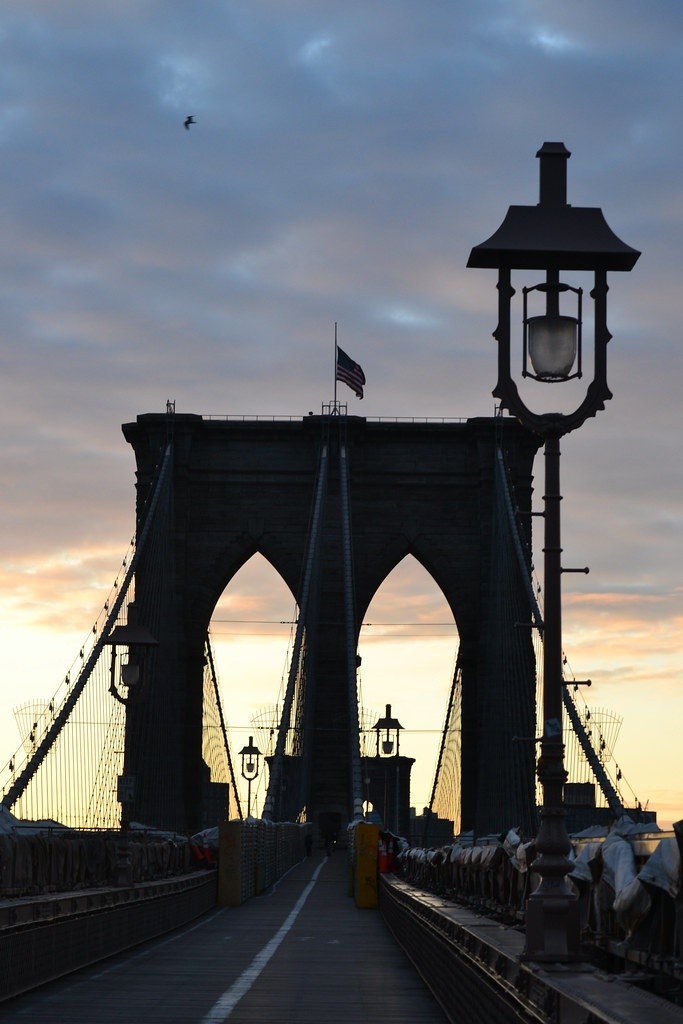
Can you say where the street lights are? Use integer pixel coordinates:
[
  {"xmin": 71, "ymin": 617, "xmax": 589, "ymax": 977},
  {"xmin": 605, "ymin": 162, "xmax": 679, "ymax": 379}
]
[
  {"xmin": 237, "ymin": 734, "xmax": 262, "ymax": 823},
  {"xmin": 372, "ymin": 703, "xmax": 405, "ymax": 883},
  {"xmin": 99, "ymin": 602, "xmax": 159, "ymax": 890},
  {"xmin": 464, "ymin": 138, "xmax": 638, "ymax": 972}
]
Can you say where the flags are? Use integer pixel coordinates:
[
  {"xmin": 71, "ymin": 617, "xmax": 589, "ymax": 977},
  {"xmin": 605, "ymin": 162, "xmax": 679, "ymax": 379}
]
[{"xmin": 335, "ymin": 346, "xmax": 366, "ymax": 401}]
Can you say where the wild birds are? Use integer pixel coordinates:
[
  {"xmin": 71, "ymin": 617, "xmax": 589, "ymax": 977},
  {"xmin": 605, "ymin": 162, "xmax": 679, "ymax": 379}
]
[{"xmin": 184, "ymin": 115, "xmax": 197, "ymax": 130}]
